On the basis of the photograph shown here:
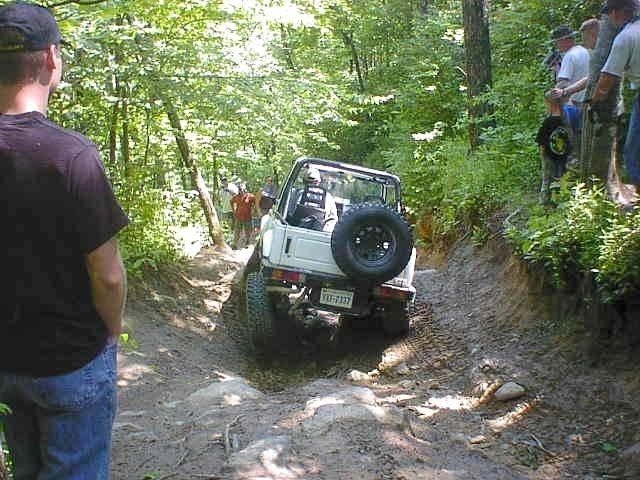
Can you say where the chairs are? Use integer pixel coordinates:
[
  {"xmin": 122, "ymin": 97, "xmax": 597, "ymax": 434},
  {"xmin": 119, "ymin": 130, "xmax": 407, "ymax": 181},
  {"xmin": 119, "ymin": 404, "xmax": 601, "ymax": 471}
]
[{"xmin": 300, "ymin": 184, "xmax": 328, "ymax": 210}]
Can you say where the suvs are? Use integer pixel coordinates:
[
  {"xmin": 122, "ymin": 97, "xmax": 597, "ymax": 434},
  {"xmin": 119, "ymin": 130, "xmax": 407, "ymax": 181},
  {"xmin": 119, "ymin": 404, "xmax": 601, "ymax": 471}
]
[{"xmin": 245, "ymin": 156, "xmax": 417, "ymax": 346}]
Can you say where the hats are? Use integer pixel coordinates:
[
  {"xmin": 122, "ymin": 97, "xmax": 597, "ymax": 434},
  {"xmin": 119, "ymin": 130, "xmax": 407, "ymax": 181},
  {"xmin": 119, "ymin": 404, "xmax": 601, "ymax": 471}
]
[
  {"xmin": 0, "ymin": 2, "xmax": 71, "ymax": 53},
  {"xmin": 551, "ymin": 25, "xmax": 578, "ymax": 42},
  {"xmin": 543, "ymin": 52, "xmax": 558, "ymax": 67},
  {"xmin": 304, "ymin": 168, "xmax": 320, "ymax": 182},
  {"xmin": 600, "ymin": 0, "xmax": 634, "ymax": 13}
]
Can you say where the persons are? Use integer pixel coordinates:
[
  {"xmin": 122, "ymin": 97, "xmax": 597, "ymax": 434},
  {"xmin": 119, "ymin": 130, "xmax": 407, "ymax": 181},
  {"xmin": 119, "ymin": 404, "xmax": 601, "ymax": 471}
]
[
  {"xmin": 255, "ymin": 176, "xmax": 278, "ymax": 217},
  {"xmin": 231, "ymin": 183, "xmax": 256, "ymax": 250},
  {"xmin": 535, "ymin": 0, "xmax": 640, "ymax": 211},
  {"xmin": 0, "ymin": 3, "xmax": 131, "ymax": 480},
  {"xmin": 287, "ymin": 167, "xmax": 339, "ymax": 221},
  {"xmin": 219, "ymin": 176, "xmax": 239, "ymax": 231}
]
